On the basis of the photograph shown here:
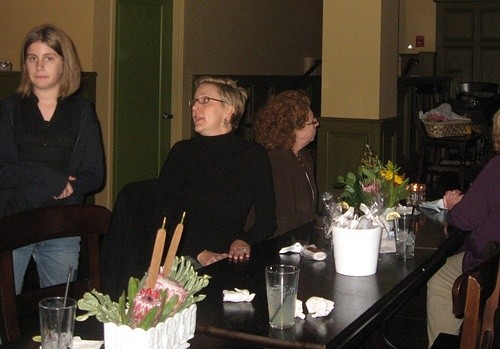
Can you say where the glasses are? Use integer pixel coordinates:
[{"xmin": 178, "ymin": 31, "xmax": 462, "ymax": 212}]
[
  {"xmin": 188, "ymin": 96, "xmax": 224, "ymax": 107},
  {"xmin": 306, "ymin": 118, "xmax": 318, "ymax": 125}
]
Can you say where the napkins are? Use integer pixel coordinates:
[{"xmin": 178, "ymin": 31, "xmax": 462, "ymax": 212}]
[
  {"xmin": 68, "ymin": 336, "xmax": 104, "ymax": 349},
  {"xmin": 279, "ymin": 242, "xmax": 307, "ymax": 253}
]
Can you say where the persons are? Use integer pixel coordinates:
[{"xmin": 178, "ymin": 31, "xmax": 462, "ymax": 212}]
[
  {"xmin": 255, "ymin": 90, "xmax": 321, "ymax": 240},
  {"xmin": 152, "ymin": 76, "xmax": 276, "ymax": 267},
  {"xmin": 427, "ymin": 110, "xmax": 500, "ymax": 349},
  {"xmin": 0, "ymin": 25, "xmax": 106, "ymax": 295}
]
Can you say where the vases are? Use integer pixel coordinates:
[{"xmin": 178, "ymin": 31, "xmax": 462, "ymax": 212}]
[
  {"xmin": 368, "ymin": 205, "xmax": 395, "ymax": 230},
  {"xmin": 103, "ymin": 304, "xmax": 197, "ymax": 349}
]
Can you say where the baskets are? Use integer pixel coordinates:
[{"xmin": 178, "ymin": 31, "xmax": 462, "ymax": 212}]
[{"xmin": 421, "ymin": 118, "xmax": 472, "ymax": 137}]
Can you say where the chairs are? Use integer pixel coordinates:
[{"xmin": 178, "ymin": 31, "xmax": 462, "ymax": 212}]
[
  {"xmin": 458, "ymin": 82, "xmax": 500, "ymax": 115},
  {"xmin": 429, "ymin": 249, "xmax": 500, "ymax": 349},
  {"xmin": 0, "ymin": 204, "xmax": 112, "ymax": 344}
]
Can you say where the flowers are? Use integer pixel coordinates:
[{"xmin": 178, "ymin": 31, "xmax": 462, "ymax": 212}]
[
  {"xmin": 78, "ymin": 213, "xmax": 212, "ymax": 329},
  {"xmin": 338, "ymin": 144, "xmax": 412, "ymax": 216}
]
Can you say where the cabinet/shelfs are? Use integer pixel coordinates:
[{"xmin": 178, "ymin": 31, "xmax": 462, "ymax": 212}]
[{"xmin": 433, "ymin": 0, "xmax": 500, "ymax": 83}]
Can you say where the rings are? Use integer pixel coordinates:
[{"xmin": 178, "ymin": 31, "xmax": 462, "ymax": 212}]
[{"xmin": 243, "ymin": 249, "xmax": 247, "ymax": 252}]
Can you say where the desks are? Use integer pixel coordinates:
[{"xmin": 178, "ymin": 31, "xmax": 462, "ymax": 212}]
[
  {"xmin": 196, "ymin": 205, "xmax": 459, "ymax": 349},
  {"xmin": 421, "ymin": 135, "xmax": 480, "ymax": 188}
]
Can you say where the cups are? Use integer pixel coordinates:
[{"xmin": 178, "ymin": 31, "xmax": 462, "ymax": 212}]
[
  {"xmin": 38, "ymin": 296, "xmax": 78, "ymax": 349},
  {"xmin": 395, "ymin": 215, "xmax": 417, "ymax": 259},
  {"xmin": 331, "ymin": 219, "xmax": 382, "ymax": 277},
  {"xmin": 265, "ymin": 264, "xmax": 300, "ymax": 329}
]
